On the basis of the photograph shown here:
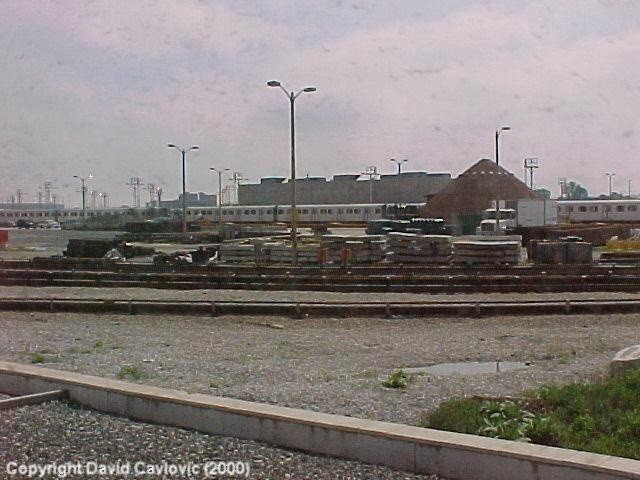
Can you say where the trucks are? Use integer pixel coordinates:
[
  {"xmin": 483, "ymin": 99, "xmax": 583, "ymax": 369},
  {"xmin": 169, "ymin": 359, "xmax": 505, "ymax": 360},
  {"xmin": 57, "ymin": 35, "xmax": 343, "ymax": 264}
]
[
  {"xmin": 17, "ymin": 219, "xmax": 38, "ymax": 229},
  {"xmin": 475, "ymin": 199, "xmax": 558, "ymax": 235}
]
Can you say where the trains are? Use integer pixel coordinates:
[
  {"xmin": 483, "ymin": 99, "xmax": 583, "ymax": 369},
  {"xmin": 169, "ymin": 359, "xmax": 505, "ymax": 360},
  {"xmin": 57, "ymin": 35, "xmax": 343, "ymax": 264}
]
[{"xmin": 0, "ymin": 200, "xmax": 640, "ymax": 223}]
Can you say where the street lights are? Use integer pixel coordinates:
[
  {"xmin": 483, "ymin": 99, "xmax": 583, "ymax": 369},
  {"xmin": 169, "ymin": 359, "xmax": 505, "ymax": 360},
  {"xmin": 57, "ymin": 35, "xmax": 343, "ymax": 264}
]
[
  {"xmin": 495, "ymin": 126, "xmax": 512, "ymax": 166},
  {"xmin": 390, "ymin": 158, "xmax": 408, "ymax": 173},
  {"xmin": 166, "ymin": 144, "xmax": 200, "ymax": 233},
  {"xmin": 72, "ymin": 175, "xmax": 85, "ymax": 209},
  {"xmin": 209, "ymin": 167, "xmax": 231, "ymax": 228},
  {"xmin": 266, "ymin": 80, "xmax": 316, "ymax": 266}
]
